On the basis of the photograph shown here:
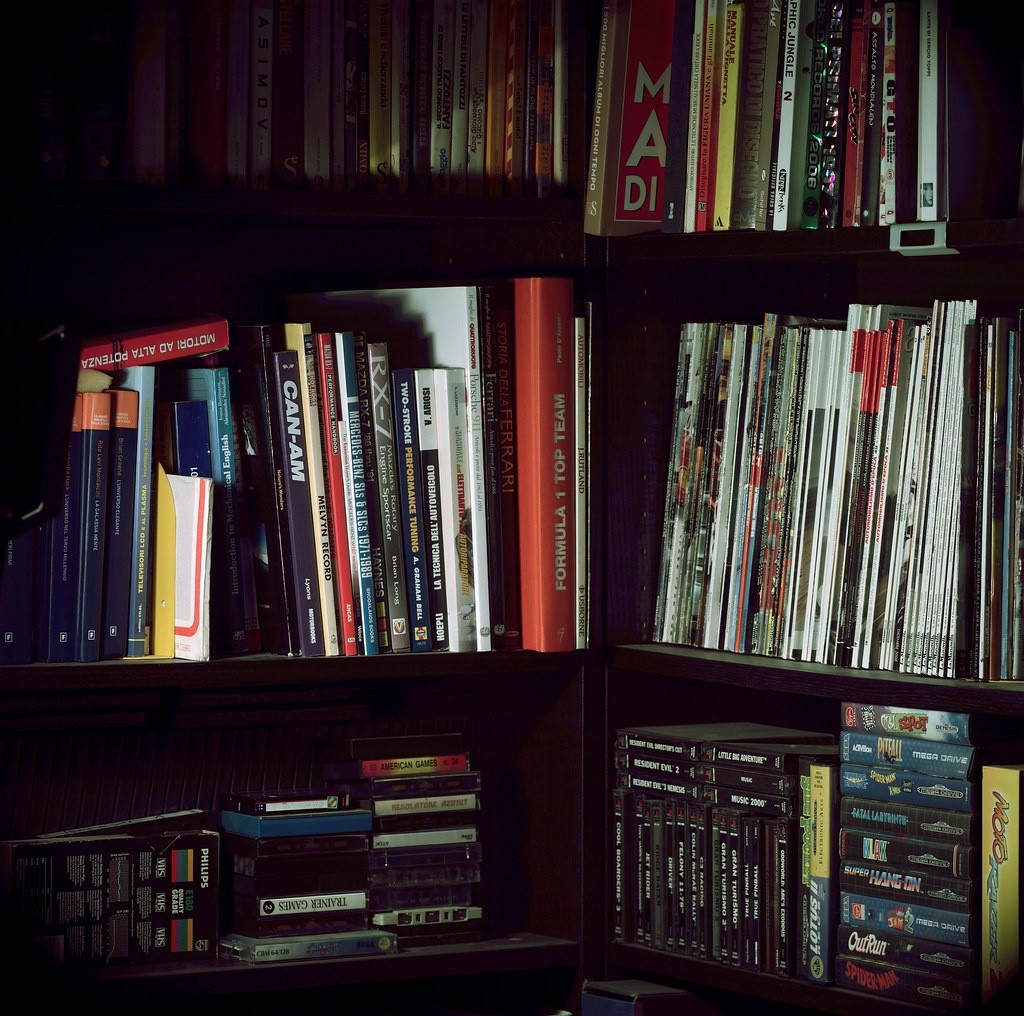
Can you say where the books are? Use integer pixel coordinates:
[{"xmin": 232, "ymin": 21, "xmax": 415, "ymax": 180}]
[
  {"xmin": 581, "ymin": 2, "xmax": 1000, "ymax": 240},
  {"xmin": 1, "ymin": 0, "xmax": 593, "ymax": 202},
  {"xmin": 652, "ymin": 298, "xmax": 1024, "ymax": 684},
  {"xmin": 0, "ymin": 273, "xmax": 591, "ymax": 667}
]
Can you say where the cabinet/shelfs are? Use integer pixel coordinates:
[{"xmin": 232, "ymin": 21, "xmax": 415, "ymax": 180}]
[{"xmin": 0, "ymin": 0, "xmax": 1024, "ymax": 1016}]
